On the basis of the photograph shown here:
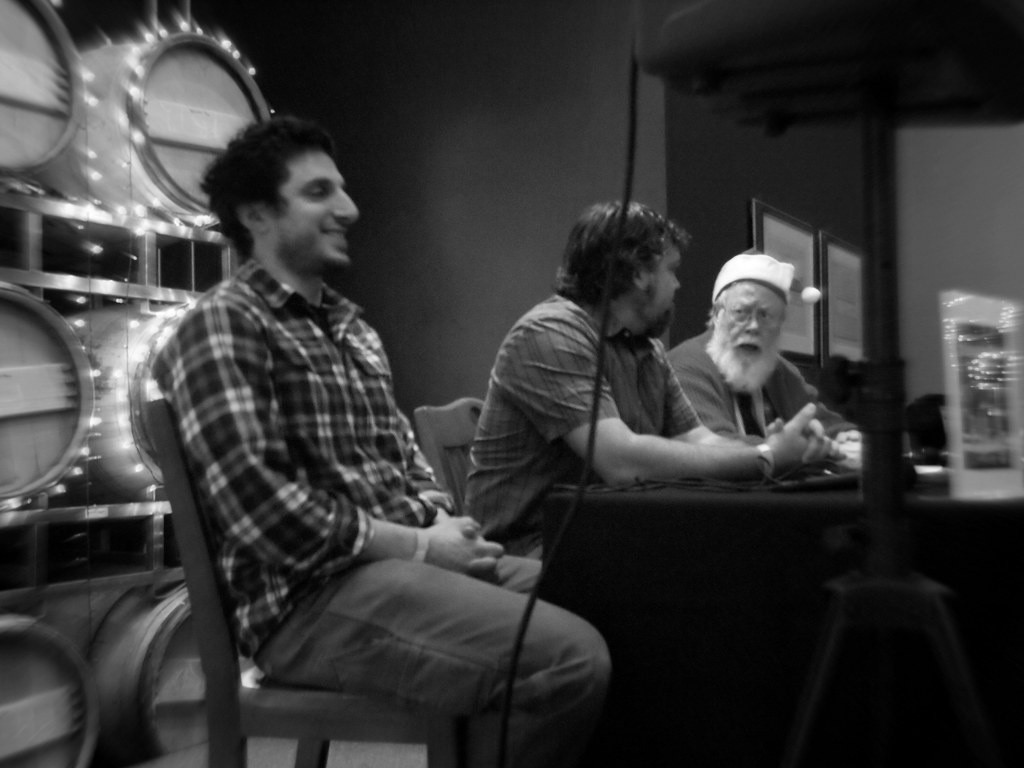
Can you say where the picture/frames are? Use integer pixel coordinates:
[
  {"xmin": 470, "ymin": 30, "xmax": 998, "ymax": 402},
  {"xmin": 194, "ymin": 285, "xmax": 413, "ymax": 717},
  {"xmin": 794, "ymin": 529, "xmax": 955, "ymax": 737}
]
[
  {"xmin": 939, "ymin": 290, "xmax": 1024, "ymax": 498},
  {"xmin": 818, "ymin": 229, "xmax": 872, "ymax": 364},
  {"xmin": 751, "ymin": 197, "xmax": 822, "ymax": 368}
]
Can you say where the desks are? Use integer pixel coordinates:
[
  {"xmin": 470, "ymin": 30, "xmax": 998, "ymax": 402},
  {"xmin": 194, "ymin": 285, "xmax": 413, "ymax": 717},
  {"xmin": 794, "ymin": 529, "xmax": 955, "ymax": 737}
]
[{"xmin": 546, "ymin": 468, "xmax": 1024, "ymax": 768}]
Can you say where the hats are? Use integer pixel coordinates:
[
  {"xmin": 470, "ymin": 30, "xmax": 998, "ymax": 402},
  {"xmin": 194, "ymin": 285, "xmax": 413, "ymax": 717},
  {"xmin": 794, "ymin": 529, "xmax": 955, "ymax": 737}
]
[{"xmin": 713, "ymin": 249, "xmax": 822, "ymax": 307}]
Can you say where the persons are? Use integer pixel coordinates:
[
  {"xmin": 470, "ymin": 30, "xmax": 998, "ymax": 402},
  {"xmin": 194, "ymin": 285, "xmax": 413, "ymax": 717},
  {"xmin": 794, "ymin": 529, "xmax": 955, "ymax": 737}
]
[
  {"xmin": 152, "ymin": 111, "xmax": 610, "ymax": 768},
  {"xmin": 665, "ymin": 247, "xmax": 862, "ymax": 473},
  {"xmin": 462, "ymin": 200, "xmax": 847, "ymax": 561}
]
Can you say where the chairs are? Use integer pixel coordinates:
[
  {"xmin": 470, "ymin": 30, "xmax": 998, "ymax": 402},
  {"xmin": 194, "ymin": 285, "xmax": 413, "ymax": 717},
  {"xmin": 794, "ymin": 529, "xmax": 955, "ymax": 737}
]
[
  {"xmin": 149, "ymin": 383, "xmax": 500, "ymax": 768},
  {"xmin": 414, "ymin": 398, "xmax": 497, "ymax": 522}
]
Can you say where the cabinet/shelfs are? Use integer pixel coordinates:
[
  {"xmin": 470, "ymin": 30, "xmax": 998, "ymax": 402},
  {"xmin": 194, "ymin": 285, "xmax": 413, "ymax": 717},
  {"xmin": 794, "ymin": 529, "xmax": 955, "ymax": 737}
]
[
  {"xmin": 0, "ymin": 500, "xmax": 211, "ymax": 768},
  {"xmin": 0, "ymin": 195, "xmax": 236, "ymax": 509}
]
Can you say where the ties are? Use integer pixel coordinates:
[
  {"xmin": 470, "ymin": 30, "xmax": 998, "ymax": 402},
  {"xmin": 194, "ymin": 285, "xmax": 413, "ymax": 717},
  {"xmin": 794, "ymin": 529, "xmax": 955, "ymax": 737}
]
[{"xmin": 738, "ymin": 389, "xmax": 763, "ymax": 438}]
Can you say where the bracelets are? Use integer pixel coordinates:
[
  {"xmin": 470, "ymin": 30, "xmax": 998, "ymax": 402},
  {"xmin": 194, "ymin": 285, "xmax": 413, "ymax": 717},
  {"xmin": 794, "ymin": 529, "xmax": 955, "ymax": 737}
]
[{"xmin": 757, "ymin": 441, "xmax": 775, "ymax": 473}]
